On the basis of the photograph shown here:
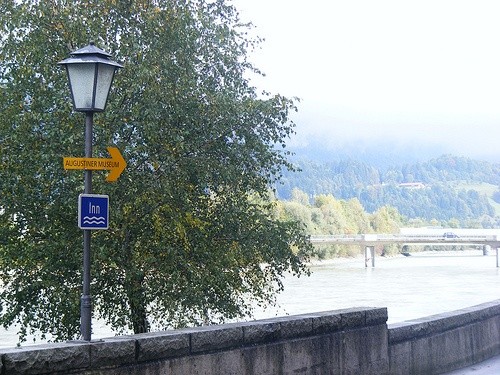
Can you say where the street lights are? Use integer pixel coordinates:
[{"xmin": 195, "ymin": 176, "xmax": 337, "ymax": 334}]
[{"xmin": 57, "ymin": 38, "xmax": 125, "ymax": 342}]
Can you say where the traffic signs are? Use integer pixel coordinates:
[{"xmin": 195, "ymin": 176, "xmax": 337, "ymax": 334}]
[{"xmin": 63, "ymin": 147, "xmax": 127, "ymax": 183}]
